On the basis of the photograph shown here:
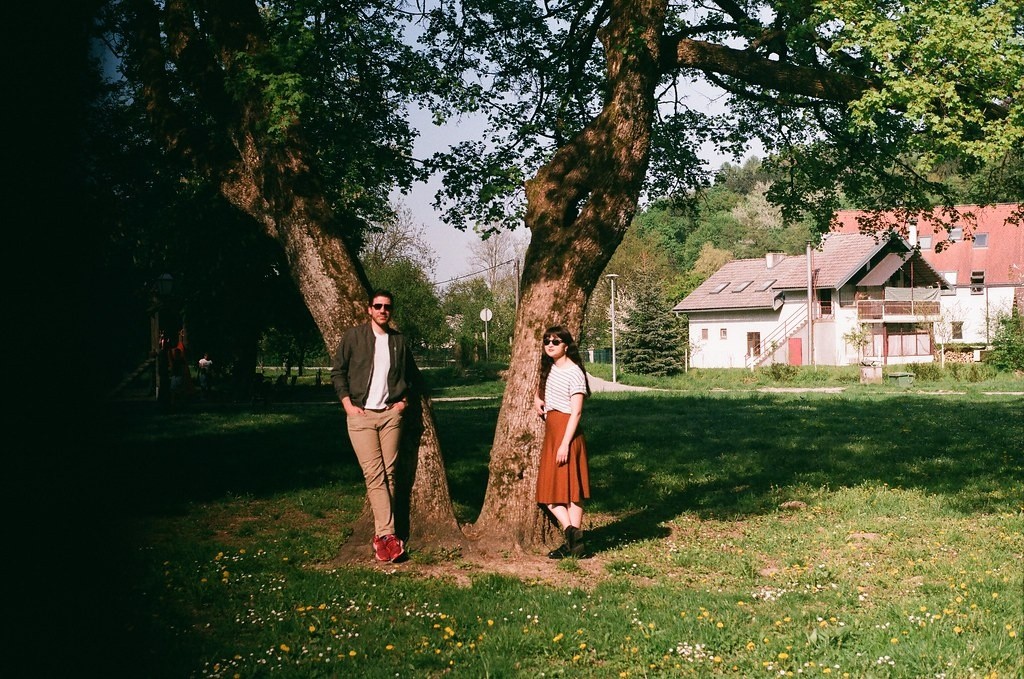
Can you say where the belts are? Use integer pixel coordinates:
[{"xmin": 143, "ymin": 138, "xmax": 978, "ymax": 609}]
[{"xmin": 367, "ymin": 406, "xmax": 387, "ymax": 413}]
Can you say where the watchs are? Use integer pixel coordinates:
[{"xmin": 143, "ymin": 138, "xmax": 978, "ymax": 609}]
[{"xmin": 401, "ymin": 401, "xmax": 409, "ymax": 407}]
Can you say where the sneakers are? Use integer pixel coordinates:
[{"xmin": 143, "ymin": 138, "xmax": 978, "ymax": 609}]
[
  {"xmin": 372, "ymin": 535, "xmax": 390, "ymax": 561},
  {"xmin": 548, "ymin": 544, "xmax": 567, "ymax": 559},
  {"xmin": 383, "ymin": 534, "xmax": 405, "ymax": 561},
  {"xmin": 565, "ymin": 525, "xmax": 587, "ymax": 559}
]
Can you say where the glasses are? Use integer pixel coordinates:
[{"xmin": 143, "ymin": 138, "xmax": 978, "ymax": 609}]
[
  {"xmin": 372, "ymin": 304, "xmax": 393, "ymax": 310},
  {"xmin": 544, "ymin": 339, "xmax": 564, "ymax": 345}
]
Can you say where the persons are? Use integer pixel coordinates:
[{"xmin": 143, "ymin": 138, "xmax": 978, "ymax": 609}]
[
  {"xmin": 160, "ymin": 330, "xmax": 322, "ymax": 401},
  {"xmin": 330, "ymin": 289, "xmax": 410, "ymax": 561},
  {"xmin": 537, "ymin": 326, "xmax": 591, "ymax": 559}
]
